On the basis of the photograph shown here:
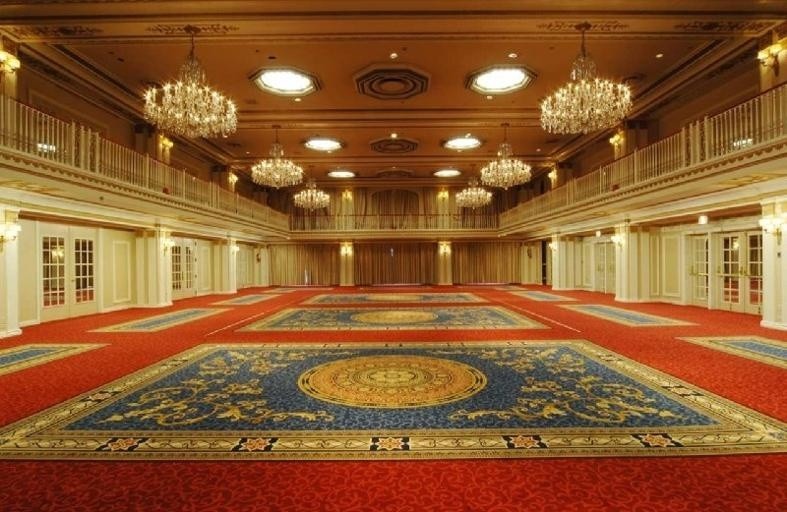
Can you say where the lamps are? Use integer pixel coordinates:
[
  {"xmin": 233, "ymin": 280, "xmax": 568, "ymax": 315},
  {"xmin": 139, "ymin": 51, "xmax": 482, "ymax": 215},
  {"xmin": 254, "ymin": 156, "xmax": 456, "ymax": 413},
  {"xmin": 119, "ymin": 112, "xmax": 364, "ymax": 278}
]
[
  {"xmin": 0, "ymin": 49, "xmax": 22, "ymax": 79},
  {"xmin": 610, "ymin": 234, "xmax": 626, "ymax": 251},
  {"xmin": 758, "ymin": 203, "xmax": 787, "ymax": 244},
  {"xmin": 292, "ymin": 164, "xmax": 331, "ymax": 212},
  {"xmin": 250, "ymin": 124, "xmax": 303, "ymax": 189},
  {"xmin": 539, "ymin": 21, "xmax": 635, "ymax": 135},
  {"xmin": 229, "ymin": 172, "xmax": 239, "ymax": 185},
  {"xmin": 159, "ymin": 238, "xmax": 174, "ymax": 257},
  {"xmin": 142, "ymin": 25, "xmax": 239, "ymax": 141},
  {"xmin": 231, "ymin": 245, "xmax": 238, "ymax": 257},
  {"xmin": 455, "ymin": 163, "xmax": 494, "ymax": 210},
  {"xmin": 1, "ymin": 220, "xmax": 21, "ymax": 256},
  {"xmin": 338, "ymin": 188, "xmax": 453, "ymax": 259},
  {"xmin": 754, "ymin": 41, "xmax": 787, "ymax": 77},
  {"xmin": 548, "ymin": 243, "xmax": 558, "ymax": 252},
  {"xmin": 607, "ymin": 133, "xmax": 625, "ymax": 153},
  {"xmin": 480, "ymin": 122, "xmax": 534, "ymax": 190},
  {"xmin": 546, "ymin": 169, "xmax": 558, "ymax": 182},
  {"xmin": 159, "ymin": 136, "xmax": 175, "ymax": 150},
  {"xmin": 256, "ymin": 254, "xmax": 261, "ymax": 263}
]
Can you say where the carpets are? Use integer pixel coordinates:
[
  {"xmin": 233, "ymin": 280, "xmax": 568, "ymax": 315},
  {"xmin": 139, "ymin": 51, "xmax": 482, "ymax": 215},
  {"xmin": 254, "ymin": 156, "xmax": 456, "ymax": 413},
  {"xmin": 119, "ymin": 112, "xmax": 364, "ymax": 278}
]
[
  {"xmin": 0, "ymin": 340, "xmax": 784, "ymax": 459},
  {"xmin": 0, "ymin": 335, "xmax": 113, "ymax": 373},
  {"xmin": 299, "ymin": 291, "xmax": 492, "ymax": 305},
  {"xmin": 210, "ymin": 285, "xmax": 300, "ymax": 308},
  {"xmin": 85, "ymin": 307, "xmax": 235, "ymax": 336},
  {"xmin": 490, "ymin": 286, "xmax": 584, "ymax": 302},
  {"xmin": 554, "ymin": 302, "xmax": 700, "ymax": 327},
  {"xmin": 678, "ymin": 332, "xmax": 783, "ymax": 373},
  {"xmin": 233, "ymin": 305, "xmax": 554, "ymax": 333}
]
